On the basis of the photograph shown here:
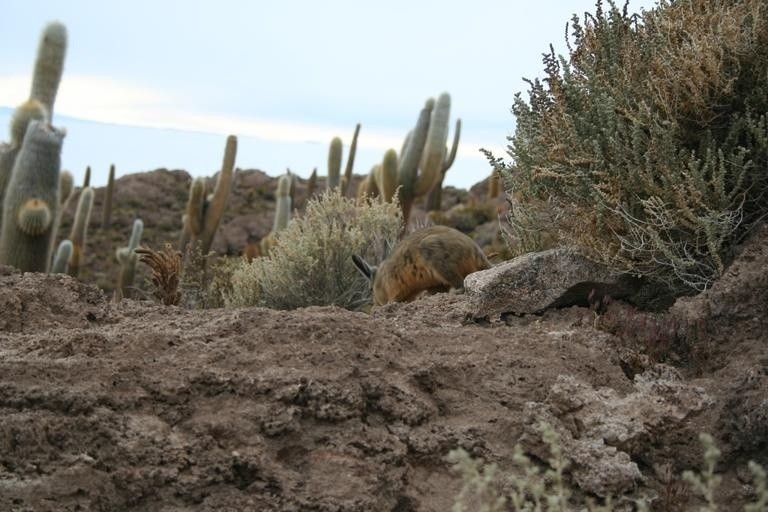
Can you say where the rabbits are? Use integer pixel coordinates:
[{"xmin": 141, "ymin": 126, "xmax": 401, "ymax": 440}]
[{"xmin": 351, "ymin": 224, "xmax": 493, "ymax": 306}]
